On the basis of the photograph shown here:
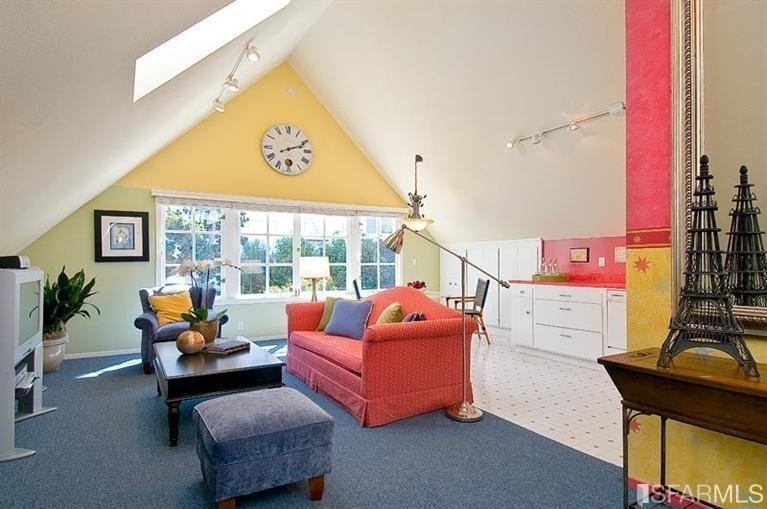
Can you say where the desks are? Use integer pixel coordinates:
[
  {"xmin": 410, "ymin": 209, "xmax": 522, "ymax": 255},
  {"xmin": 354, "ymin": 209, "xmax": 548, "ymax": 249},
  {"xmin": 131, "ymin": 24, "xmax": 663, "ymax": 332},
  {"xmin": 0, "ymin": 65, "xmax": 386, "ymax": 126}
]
[{"xmin": 596, "ymin": 341, "xmax": 766, "ymax": 507}]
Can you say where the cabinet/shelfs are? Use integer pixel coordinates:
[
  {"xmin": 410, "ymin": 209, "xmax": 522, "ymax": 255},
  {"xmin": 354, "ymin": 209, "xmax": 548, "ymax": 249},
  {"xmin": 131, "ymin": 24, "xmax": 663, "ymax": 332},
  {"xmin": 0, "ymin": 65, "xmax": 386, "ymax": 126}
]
[{"xmin": 438, "ymin": 239, "xmax": 627, "ymax": 371}]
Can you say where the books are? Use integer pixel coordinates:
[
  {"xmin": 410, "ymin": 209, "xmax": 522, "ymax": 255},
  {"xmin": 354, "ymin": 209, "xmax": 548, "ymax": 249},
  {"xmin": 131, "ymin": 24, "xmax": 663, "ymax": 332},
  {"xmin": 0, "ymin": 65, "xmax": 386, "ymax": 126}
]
[{"xmin": 207, "ymin": 340, "xmax": 250, "ymax": 356}]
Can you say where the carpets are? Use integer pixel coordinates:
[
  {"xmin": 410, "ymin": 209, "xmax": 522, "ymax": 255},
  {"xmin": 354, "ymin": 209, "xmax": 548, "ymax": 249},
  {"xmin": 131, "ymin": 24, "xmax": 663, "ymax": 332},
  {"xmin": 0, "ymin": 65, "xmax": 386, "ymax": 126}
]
[{"xmin": 16, "ymin": 326, "xmax": 688, "ymax": 508}]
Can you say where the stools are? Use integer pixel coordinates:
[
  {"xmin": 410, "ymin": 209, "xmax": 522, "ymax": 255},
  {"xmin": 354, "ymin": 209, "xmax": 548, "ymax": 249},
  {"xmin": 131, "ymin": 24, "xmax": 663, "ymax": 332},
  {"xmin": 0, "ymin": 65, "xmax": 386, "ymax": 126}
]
[{"xmin": 196, "ymin": 388, "xmax": 333, "ymax": 509}]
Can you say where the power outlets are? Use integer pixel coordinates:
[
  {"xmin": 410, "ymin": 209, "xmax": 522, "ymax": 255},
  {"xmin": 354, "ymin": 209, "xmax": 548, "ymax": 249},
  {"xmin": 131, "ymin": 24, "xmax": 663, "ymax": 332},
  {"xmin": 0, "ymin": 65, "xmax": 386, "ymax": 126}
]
[{"xmin": 598, "ymin": 257, "xmax": 605, "ymax": 268}]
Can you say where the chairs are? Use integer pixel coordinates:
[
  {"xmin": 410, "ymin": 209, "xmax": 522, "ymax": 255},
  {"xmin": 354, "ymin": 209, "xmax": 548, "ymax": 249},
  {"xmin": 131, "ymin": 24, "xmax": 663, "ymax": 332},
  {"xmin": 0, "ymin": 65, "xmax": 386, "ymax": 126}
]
[{"xmin": 444, "ymin": 276, "xmax": 492, "ymax": 344}]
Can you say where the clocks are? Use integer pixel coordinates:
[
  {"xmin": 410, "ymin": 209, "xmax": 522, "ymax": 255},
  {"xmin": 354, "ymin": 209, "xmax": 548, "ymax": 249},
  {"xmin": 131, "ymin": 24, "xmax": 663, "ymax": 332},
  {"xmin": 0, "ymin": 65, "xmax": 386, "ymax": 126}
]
[{"xmin": 256, "ymin": 119, "xmax": 318, "ymax": 176}]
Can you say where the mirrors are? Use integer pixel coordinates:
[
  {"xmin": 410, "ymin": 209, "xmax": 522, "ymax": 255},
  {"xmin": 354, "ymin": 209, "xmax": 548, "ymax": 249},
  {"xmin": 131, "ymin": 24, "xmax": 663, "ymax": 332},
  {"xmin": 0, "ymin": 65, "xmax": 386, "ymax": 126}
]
[{"xmin": 678, "ymin": 4, "xmax": 765, "ymax": 327}]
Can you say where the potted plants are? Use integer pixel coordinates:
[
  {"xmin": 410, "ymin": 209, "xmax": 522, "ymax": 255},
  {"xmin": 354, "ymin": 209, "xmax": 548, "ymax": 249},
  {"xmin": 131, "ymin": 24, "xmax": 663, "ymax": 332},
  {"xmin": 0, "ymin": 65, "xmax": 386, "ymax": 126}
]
[
  {"xmin": 175, "ymin": 288, "xmax": 231, "ymax": 348},
  {"xmin": 29, "ymin": 267, "xmax": 102, "ymax": 373}
]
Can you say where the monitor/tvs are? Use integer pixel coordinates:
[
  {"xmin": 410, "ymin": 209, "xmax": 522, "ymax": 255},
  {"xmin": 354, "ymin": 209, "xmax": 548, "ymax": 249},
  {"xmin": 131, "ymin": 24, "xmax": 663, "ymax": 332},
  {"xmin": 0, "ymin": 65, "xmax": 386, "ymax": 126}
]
[{"xmin": 0, "ymin": 269, "xmax": 45, "ymax": 366}]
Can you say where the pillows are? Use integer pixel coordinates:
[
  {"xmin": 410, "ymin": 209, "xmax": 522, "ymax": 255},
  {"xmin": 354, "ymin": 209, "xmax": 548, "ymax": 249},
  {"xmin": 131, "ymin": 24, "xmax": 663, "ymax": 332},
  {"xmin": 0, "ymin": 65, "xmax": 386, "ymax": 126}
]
[
  {"xmin": 375, "ymin": 300, "xmax": 401, "ymax": 334},
  {"xmin": 401, "ymin": 310, "xmax": 426, "ymax": 328},
  {"xmin": 330, "ymin": 299, "xmax": 372, "ymax": 341},
  {"xmin": 318, "ymin": 296, "xmax": 333, "ymax": 332},
  {"xmin": 147, "ymin": 291, "xmax": 196, "ymax": 321}
]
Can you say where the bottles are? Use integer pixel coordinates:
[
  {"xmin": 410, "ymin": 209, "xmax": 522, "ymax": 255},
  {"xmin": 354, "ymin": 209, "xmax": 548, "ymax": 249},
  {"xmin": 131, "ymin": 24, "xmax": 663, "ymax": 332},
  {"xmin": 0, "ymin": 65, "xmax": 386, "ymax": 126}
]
[{"xmin": 541, "ymin": 255, "xmax": 559, "ymax": 274}]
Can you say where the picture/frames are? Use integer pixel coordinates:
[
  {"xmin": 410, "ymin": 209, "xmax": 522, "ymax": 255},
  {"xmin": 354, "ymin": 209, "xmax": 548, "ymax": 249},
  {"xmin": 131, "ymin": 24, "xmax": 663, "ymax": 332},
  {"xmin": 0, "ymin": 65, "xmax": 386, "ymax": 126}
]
[{"xmin": 91, "ymin": 211, "xmax": 148, "ymax": 262}]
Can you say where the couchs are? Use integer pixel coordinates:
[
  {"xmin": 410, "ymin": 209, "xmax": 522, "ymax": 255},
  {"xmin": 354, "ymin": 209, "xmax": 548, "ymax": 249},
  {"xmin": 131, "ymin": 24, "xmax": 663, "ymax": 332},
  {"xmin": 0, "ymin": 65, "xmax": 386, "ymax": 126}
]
[
  {"xmin": 285, "ymin": 285, "xmax": 479, "ymax": 429},
  {"xmin": 133, "ymin": 283, "xmax": 234, "ymax": 367}
]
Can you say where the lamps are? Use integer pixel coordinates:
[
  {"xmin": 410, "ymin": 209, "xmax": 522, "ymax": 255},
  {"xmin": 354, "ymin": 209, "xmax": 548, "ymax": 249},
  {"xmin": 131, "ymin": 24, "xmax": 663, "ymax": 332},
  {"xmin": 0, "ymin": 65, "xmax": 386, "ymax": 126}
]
[
  {"xmin": 504, "ymin": 122, "xmax": 581, "ymax": 151},
  {"xmin": 400, "ymin": 153, "xmax": 436, "ymax": 235},
  {"xmin": 211, "ymin": 42, "xmax": 260, "ymax": 114},
  {"xmin": 295, "ymin": 258, "xmax": 332, "ymax": 303}
]
